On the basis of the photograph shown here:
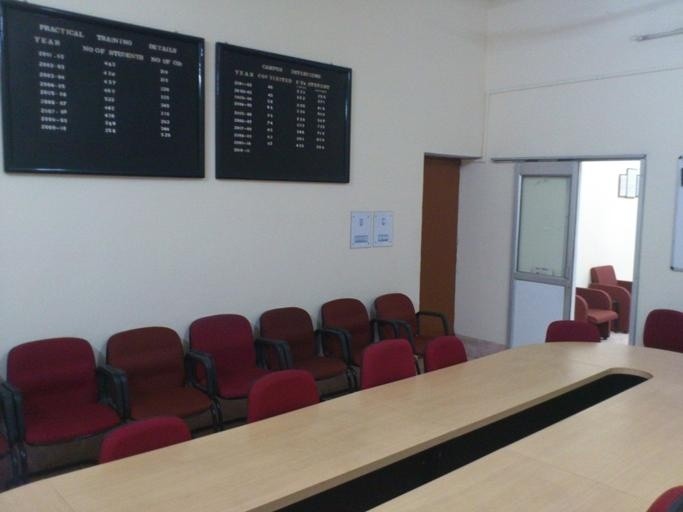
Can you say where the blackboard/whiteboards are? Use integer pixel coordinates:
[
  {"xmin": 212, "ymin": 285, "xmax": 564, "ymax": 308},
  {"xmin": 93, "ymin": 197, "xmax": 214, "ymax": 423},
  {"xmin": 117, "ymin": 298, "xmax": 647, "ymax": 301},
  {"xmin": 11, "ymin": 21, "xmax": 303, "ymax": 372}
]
[{"xmin": 671, "ymin": 157, "xmax": 683, "ymax": 271}]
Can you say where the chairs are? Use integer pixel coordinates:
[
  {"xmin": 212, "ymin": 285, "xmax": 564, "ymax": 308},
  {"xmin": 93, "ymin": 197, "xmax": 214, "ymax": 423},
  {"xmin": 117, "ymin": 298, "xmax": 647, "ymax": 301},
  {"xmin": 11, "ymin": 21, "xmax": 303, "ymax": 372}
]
[
  {"xmin": 641, "ymin": 308, "xmax": 683, "ymax": 354},
  {"xmin": 0, "ymin": 386, "xmax": 21, "ymax": 491},
  {"xmin": 372, "ymin": 292, "xmax": 449, "ymax": 356},
  {"xmin": 98, "ymin": 416, "xmax": 192, "ymax": 467},
  {"xmin": 100, "ymin": 326, "xmax": 217, "ymax": 450},
  {"xmin": 588, "ymin": 265, "xmax": 632, "ymax": 335},
  {"xmin": 547, "ymin": 320, "xmax": 603, "ymax": 344},
  {"xmin": 5, "ymin": 334, "xmax": 125, "ymax": 487},
  {"xmin": 247, "ymin": 368, "xmax": 322, "ymax": 425},
  {"xmin": 187, "ymin": 311, "xmax": 286, "ymax": 439},
  {"xmin": 359, "ymin": 338, "xmax": 418, "ymax": 391},
  {"xmin": 645, "ymin": 486, "xmax": 683, "ymax": 512},
  {"xmin": 258, "ymin": 306, "xmax": 354, "ymax": 399},
  {"xmin": 319, "ymin": 296, "xmax": 402, "ymax": 395},
  {"xmin": 421, "ymin": 334, "xmax": 468, "ymax": 372},
  {"xmin": 575, "ymin": 287, "xmax": 618, "ymax": 341}
]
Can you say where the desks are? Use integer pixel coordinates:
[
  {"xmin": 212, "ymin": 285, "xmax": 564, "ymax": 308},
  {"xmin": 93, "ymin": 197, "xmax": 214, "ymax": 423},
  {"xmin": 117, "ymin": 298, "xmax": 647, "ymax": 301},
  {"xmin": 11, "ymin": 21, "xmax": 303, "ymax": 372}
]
[{"xmin": 0, "ymin": 341, "xmax": 681, "ymax": 511}]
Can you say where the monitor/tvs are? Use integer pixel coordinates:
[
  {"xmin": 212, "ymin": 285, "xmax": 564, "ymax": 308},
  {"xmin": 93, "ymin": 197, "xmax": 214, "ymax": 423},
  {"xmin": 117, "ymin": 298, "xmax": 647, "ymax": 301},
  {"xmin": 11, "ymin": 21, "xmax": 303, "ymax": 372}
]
[
  {"xmin": 214, "ymin": 42, "xmax": 353, "ymax": 184},
  {"xmin": 2, "ymin": 2, "xmax": 206, "ymax": 179}
]
